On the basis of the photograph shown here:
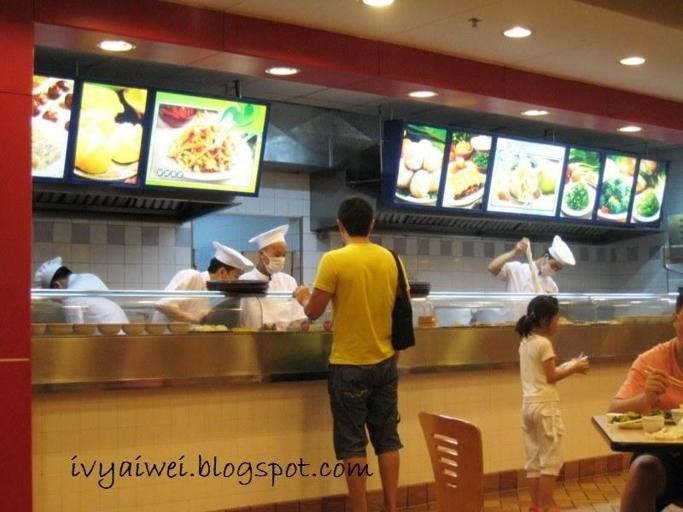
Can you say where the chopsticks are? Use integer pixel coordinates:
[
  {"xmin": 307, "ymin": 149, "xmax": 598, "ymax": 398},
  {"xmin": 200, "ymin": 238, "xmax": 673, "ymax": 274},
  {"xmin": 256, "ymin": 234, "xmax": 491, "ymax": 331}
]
[{"xmin": 642, "ymin": 365, "xmax": 682, "ymax": 389}]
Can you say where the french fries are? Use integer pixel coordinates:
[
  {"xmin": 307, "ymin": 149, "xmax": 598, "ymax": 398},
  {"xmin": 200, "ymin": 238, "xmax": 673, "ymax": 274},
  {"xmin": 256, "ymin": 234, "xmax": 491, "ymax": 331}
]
[{"xmin": 168, "ymin": 124, "xmax": 237, "ymax": 172}]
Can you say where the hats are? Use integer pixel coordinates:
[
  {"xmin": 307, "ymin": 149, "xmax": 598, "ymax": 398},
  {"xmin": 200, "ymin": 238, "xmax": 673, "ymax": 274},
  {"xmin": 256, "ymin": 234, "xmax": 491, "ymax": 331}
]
[
  {"xmin": 549, "ymin": 235, "xmax": 575, "ymax": 266},
  {"xmin": 248, "ymin": 224, "xmax": 289, "ymax": 250},
  {"xmin": 213, "ymin": 241, "xmax": 254, "ymax": 273},
  {"xmin": 33, "ymin": 256, "xmax": 63, "ymax": 288}
]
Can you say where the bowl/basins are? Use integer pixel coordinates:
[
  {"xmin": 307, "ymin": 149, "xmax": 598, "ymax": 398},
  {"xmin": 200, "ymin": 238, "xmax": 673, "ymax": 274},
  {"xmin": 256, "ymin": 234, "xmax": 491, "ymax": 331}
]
[{"xmin": 31, "ymin": 321, "xmax": 191, "ymax": 338}]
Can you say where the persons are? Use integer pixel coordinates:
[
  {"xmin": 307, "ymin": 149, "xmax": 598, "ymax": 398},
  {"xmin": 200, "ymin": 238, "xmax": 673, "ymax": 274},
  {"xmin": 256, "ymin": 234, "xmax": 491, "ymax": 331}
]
[
  {"xmin": 610, "ymin": 283, "xmax": 683, "ymax": 512},
  {"xmin": 513, "ymin": 291, "xmax": 593, "ymax": 512},
  {"xmin": 488, "ymin": 233, "xmax": 579, "ymax": 295},
  {"xmin": 292, "ymin": 196, "xmax": 417, "ymax": 512},
  {"xmin": 235, "ymin": 221, "xmax": 311, "ymax": 331},
  {"xmin": 149, "ymin": 240, "xmax": 255, "ymax": 335},
  {"xmin": 33, "ymin": 255, "xmax": 132, "ymax": 336}
]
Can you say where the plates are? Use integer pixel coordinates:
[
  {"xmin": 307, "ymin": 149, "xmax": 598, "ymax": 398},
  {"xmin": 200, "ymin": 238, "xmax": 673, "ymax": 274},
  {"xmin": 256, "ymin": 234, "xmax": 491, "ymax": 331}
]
[
  {"xmin": 395, "ymin": 154, "xmax": 443, "ymax": 206},
  {"xmin": 615, "ymin": 423, "xmax": 642, "ymax": 429},
  {"xmin": 158, "ymin": 124, "xmax": 252, "ymax": 183},
  {"xmin": 74, "ymin": 161, "xmax": 139, "ymax": 182},
  {"xmin": 443, "ymin": 159, "xmax": 485, "ymax": 208},
  {"xmin": 631, "ymin": 194, "xmax": 660, "ymax": 224},
  {"xmin": 30, "ymin": 114, "xmax": 68, "ymax": 178},
  {"xmin": 408, "ymin": 281, "xmax": 430, "ymax": 294},
  {"xmin": 204, "ymin": 278, "xmax": 269, "ymax": 293},
  {"xmin": 559, "ymin": 182, "xmax": 596, "ymax": 218},
  {"xmin": 597, "ymin": 210, "xmax": 629, "ymax": 222}
]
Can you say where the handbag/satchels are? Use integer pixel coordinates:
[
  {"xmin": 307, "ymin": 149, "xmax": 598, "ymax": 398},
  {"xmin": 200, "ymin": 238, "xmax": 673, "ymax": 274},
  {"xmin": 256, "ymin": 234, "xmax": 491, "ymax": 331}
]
[{"xmin": 392, "ymin": 276, "xmax": 415, "ymax": 350}]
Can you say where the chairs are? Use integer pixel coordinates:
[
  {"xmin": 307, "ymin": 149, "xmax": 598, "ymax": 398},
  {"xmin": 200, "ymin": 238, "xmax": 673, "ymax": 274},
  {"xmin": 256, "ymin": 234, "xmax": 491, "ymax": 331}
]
[{"xmin": 416, "ymin": 410, "xmax": 485, "ymax": 512}]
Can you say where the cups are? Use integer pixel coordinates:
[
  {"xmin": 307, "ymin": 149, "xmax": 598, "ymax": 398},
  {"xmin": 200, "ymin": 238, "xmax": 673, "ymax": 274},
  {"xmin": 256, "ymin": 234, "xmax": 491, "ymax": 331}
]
[
  {"xmin": 669, "ymin": 409, "xmax": 682, "ymax": 426},
  {"xmin": 641, "ymin": 415, "xmax": 665, "ymax": 435},
  {"xmin": 417, "ymin": 315, "xmax": 436, "ymax": 326}
]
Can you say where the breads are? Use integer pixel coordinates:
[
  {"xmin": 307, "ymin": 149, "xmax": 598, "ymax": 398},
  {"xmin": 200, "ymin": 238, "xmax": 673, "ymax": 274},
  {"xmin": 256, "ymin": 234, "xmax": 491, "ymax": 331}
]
[
  {"xmin": 450, "ymin": 172, "xmax": 485, "ymax": 199},
  {"xmin": 398, "ymin": 138, "xmax": 444, "ymax": 197},
  {"xmin": 74, "ymin": 84, "xmax": 147, "ymax": 175}
]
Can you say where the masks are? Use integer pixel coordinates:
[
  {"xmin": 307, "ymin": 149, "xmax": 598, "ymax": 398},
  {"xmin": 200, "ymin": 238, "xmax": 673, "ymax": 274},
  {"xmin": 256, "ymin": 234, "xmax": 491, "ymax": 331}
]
[
  {"xmin": 262, "ymin": 254, "xmax": 285, "ymax": 275},
  {"xmin": 541, "ymin": 263, "xmax": 555, "ymax": 277}
]
